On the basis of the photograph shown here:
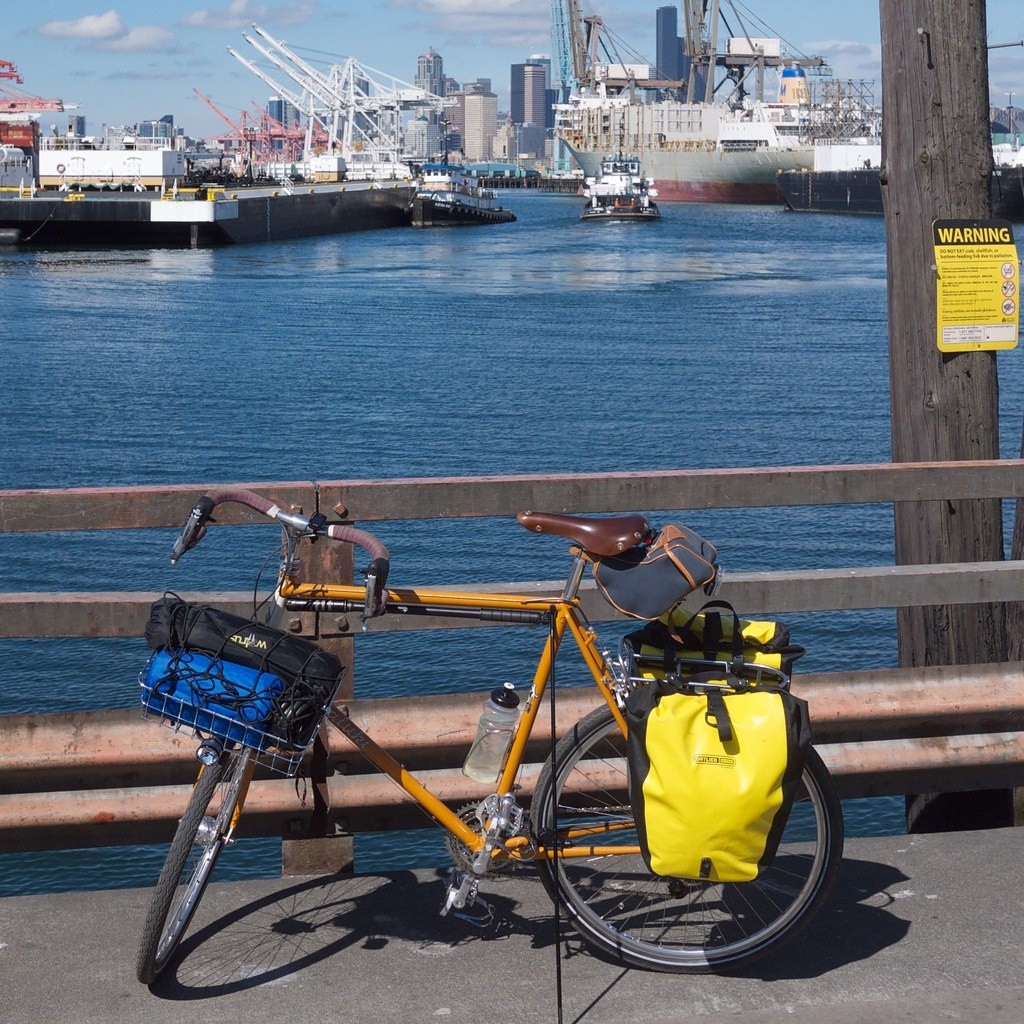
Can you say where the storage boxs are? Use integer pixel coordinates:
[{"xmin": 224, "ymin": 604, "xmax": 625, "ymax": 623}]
[
  {"xmin": 725, "ymin": 37, "xmax": 780, "ymax": 58},
  {"xmin": 605, "ymin": 64, "xmax": 650, "ymax": 80},
  {"xmin": 310, "ymin": 155, "xmax": 346, "ymax": 181},
  {"xmin": 39, "ymin": 150, "xmax": 184, "ymax": 177},
  {"xmin": 0, "ymin": 121, "xmax": 39, "ymax": 148}
]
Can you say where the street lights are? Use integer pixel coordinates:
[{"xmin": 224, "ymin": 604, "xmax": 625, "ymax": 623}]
[{"xmin": 1003, "ymin": 90, "xmax": 1014, "ymax": 132}]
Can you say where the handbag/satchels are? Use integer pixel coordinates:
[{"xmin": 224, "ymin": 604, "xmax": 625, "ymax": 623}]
[{"xmin": 624, "ymin": 600, "xmax": 811, "ymax": 883}]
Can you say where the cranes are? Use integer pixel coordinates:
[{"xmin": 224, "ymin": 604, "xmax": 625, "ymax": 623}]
[
  {"xmin": 0, "ymin": 56, "xmax": 83, "ymax": 122},
  {"xmin": 678, "ymin": 0, "xmax": 826, "ymax": 105},
  {"xmin": 561, "ymin": 0, "xmax": 685, "ymax": 105},
  {"xmin": 188, "ymin": 22, "xmax": 464, "ymax": 182}
]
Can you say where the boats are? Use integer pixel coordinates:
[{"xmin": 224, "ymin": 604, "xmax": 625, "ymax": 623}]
[
  {"xmin": 577, "ymin": 121, "xmax": 663, "ymax": 223},
  {"xmin": 774, "ymin": 116, "xmax": 1023, "ymax": 220},
  {"xmin": 551, "ymin": 56, "xmax": 888, "ymax": 204},
  {"xmin": 407, "ymin": 114, "xmax": 516, "ymax": 228}
]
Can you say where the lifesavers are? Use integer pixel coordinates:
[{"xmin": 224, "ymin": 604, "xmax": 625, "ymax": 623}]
[
  {"xmin": 57, "ymin": 164, "xmax": 65, "ymax": 173},
  {"xmin": 448, "ymin": 207, "xmax": 514, "ymax": 222}
]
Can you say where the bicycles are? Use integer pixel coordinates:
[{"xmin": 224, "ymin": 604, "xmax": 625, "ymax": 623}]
[{"xmin": 134, "ymin": 485, "xmax": 845, "ymax": 1023}]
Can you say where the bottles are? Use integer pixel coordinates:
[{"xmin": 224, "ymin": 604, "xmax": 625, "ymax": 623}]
[{"xmin": 463, "ymin": 681, "xmax": 521, "ymax": 784}]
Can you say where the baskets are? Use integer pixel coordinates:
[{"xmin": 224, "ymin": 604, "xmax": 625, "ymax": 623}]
[{"xmin": 138, "ymin": 650, "xmax": 346, "ymax": 777}]
